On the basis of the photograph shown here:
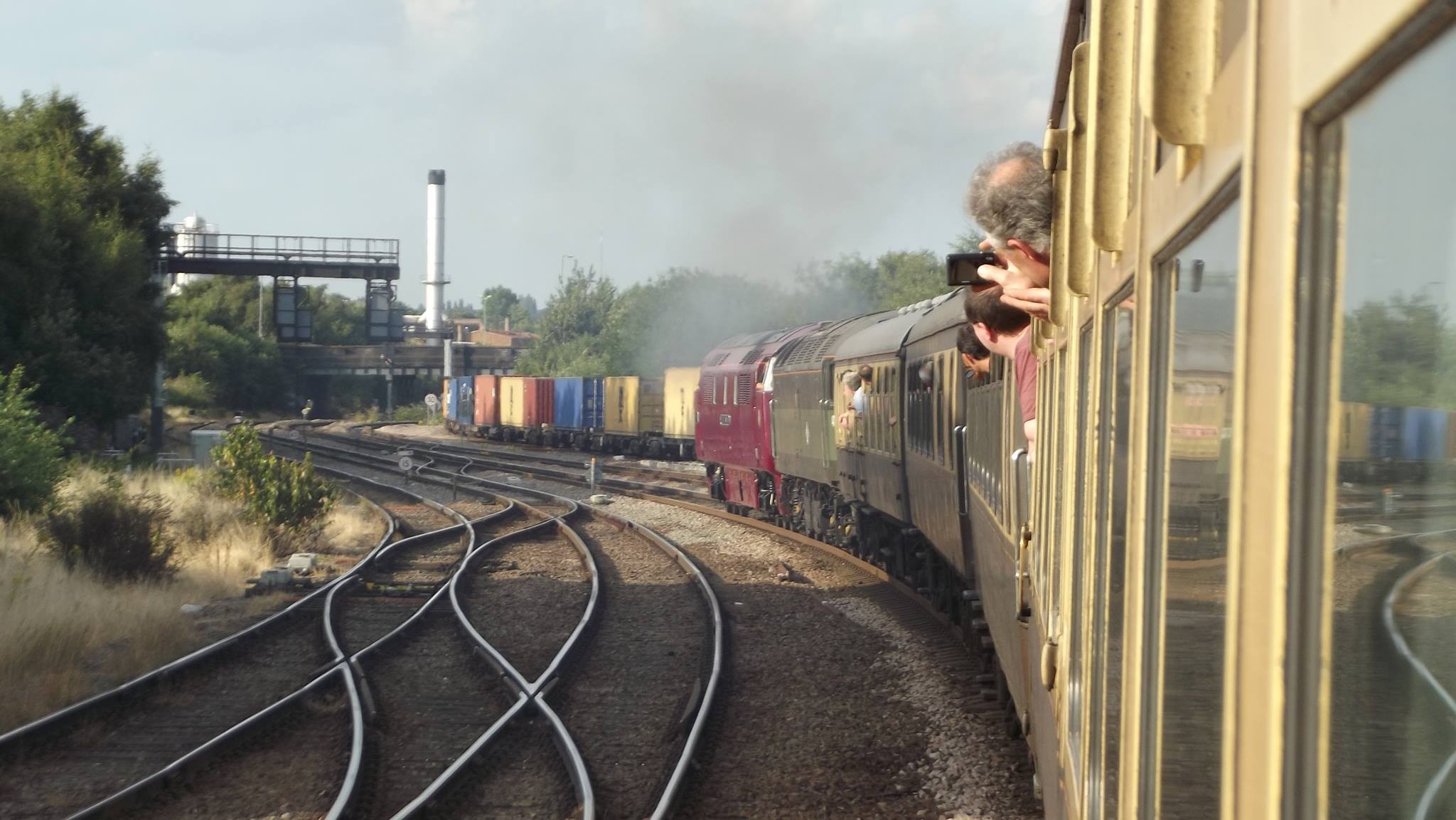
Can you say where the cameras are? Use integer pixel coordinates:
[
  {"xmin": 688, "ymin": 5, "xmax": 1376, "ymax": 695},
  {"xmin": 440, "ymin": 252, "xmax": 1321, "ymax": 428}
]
[{"xmin": 945, "ymin": 251, "xmax": 996, "ymax": 287}]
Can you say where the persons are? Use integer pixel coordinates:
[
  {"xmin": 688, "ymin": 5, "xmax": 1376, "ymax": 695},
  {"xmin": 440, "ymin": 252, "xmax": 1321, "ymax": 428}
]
[
  {"xmin": 918, "ymin": 360, "xmax": 934, "ymax": 388},
  {"xmin": 967, "ymin": 140, "xmax": 1053, "ymax": 321},
  {"xmin": 859, "ymin": 364, "xmax": 872, "ymax": 386},
  {"xmin": 842, "ymin": 372, "xmax": 863, "ymax": 421},
  {"xmin": 964, "ymin": 285, "xmax": 1037, "ymax": 444},
  {"xmin": 957, "ymin": 323, "xmax": 990, "ymax": 375}
]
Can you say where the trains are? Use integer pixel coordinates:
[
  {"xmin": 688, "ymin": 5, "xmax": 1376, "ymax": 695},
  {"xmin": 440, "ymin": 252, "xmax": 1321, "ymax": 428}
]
[{"xmin": 440, "ymin": 0, "xmax": 1456, "ymax": 820}]
